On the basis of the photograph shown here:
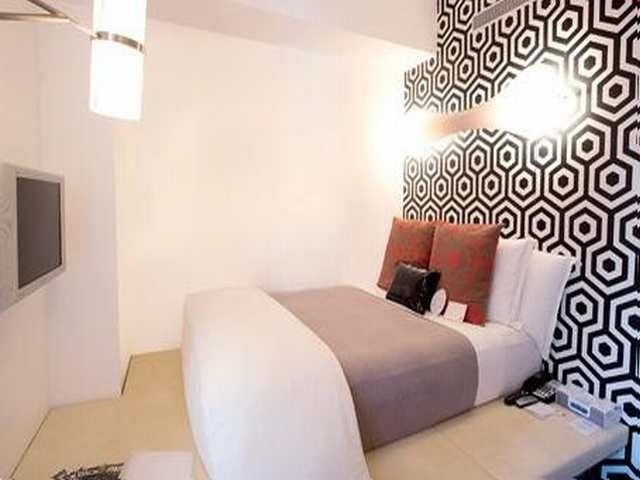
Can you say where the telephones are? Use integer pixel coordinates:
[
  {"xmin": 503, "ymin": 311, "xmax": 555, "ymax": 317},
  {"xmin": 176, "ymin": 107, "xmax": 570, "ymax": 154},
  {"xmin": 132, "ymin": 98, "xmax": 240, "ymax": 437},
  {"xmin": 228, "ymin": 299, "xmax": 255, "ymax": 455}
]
[{"xmin": 520, "ymin": 371, "xmax": 565, "ymax": 405}]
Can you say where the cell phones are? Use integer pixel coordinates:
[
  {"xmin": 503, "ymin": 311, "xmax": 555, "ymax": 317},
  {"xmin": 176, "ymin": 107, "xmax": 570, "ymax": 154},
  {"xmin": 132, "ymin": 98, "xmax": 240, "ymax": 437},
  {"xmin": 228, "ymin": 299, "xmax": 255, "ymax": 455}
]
[{"xmin": 516, "ymin": 394, "xmax": 538, "ymax": 408}]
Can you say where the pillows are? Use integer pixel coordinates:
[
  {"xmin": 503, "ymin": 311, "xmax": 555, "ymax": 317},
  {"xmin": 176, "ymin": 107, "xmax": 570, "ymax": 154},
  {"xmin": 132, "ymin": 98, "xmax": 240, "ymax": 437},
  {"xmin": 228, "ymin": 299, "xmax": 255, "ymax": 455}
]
[
  {"xmin": 486, "ymin": 235, "xmax": 538, "ymax": 329},
  {"xmin": 375, "ymin": 216, "xmax": 435, "ymax": 291},
  {"xmin": 517, "ymin": 250, "xmax": 578, "ymax": 361},
  {"xmin": 429, "ymin": 220, "xmax": 507, "ymax": 327},
  {"xmin": 385, "ymin": 260, "xmax": 441, "ymax": 315}
]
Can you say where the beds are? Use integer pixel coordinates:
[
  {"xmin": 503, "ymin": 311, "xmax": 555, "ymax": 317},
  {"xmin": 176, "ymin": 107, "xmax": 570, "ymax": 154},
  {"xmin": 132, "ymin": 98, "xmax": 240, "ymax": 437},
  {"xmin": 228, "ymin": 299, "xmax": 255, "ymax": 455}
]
[{"xmin": 180, "ymin": 283, "xmax": 579, "ymax": 480}]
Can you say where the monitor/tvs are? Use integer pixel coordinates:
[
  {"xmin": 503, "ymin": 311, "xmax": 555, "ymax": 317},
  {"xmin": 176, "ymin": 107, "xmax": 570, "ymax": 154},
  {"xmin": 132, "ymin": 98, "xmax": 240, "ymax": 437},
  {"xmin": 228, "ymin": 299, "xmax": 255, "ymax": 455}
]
[{"xmin": 1, "ymin": 158, "xmax": 70, "ymax": 312}]
